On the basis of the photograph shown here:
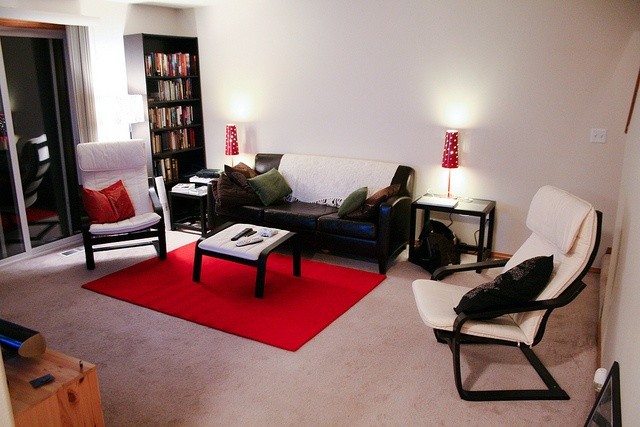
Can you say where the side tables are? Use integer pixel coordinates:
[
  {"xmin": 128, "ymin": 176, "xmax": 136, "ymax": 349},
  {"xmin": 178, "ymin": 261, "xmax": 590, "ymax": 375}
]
[
  {"xmin": 408, "ymin": 191, "xmax": 496, "ymax": 273},
  {"xmin": 166, "ymin": 177, "xmax": 212, "ymax": 237}
]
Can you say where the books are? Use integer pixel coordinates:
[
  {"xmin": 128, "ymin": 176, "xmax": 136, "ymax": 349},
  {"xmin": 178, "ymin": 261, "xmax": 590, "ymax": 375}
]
[
  {"xmin": 146, "ymin": 77, "xmax": 194, "ymax": 102},
  {"xmin": 144, "ymin": 51, "xmax": 198, "ymax": 77},
  {"xmin": 148, "ymin": 104, "xmax": 196, "ymax": 130},
  {"xmin": 150, "ymin": 127, "xmax": 199, "ymax": 153},
  {"xmin": 153, "ymin": 156, "xmax": 181, "ymax": 182}
]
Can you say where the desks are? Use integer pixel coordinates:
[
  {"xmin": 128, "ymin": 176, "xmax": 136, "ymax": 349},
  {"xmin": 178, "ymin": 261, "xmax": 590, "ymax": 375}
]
[{"xmin": 0, "ymin": 316, "xmax": 106, "ymax": 427}]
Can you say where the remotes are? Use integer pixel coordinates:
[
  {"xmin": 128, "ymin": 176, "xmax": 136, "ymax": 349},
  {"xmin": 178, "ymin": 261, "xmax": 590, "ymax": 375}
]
[
  {"xmin": 231, "ymin": 228, "xmax": 253, "ymax": 241},
  {"xmin": 235, "ymin": 236, "xmax": 264, "ymax": 247}
]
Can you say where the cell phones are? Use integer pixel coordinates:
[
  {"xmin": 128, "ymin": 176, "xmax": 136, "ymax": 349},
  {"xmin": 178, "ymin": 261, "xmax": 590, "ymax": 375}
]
[
  {"xmin": 243, "ymin": 230, "xmax": 257, "ymax": 236},
  {"xmin": 30, "ymin": 373, "xmax": 56, "ymax": 388}
]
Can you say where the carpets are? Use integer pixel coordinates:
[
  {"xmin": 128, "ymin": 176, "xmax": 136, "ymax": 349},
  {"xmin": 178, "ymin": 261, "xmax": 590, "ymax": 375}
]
[{"xmin": 81, "ymin": 237, "xmax": 388, "ymax": 353}]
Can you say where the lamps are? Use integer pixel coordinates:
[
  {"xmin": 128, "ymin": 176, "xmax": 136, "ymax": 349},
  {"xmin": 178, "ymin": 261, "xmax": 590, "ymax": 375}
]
[
  {"xmin": 439, "ymin": 129, "xmax": 460, "ymax": 197},
  {"xmin": 125, "ymin": 94, "xmax": 146, "ymax": 139},
  {"xmin": 224, "ymin": 122, "xmax": 240, "ymax": 169}
]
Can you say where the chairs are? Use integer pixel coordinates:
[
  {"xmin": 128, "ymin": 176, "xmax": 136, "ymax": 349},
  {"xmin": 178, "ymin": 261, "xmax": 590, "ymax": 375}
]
[
  {"xmin": 410, "ymin": 185, "xmax": 604, "ymax": 403},
  {"xmin": 74, "ymin": 139, "xmax": 167, "ymax": 269},
  {"xmin": 2, "ymin": 132, "xmax": 57, "ymax": 243}
]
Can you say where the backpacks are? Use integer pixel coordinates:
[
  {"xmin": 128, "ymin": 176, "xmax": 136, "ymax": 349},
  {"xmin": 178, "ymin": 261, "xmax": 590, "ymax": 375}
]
[{"xmin": 412, "ymin": 219, "xmax": 469, "ymax": 278}]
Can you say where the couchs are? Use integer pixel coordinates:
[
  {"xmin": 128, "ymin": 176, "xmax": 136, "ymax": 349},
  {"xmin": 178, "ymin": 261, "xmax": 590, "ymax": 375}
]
[{"xmin": 205, "ymin": 151, "xmax": 416, "ymax": 275}]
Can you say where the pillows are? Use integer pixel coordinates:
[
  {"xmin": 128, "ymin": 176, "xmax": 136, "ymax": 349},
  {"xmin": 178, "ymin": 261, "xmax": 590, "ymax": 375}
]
[
  {"xmin": 245, "ymin": 167, "xmax": 293, "ymax": 207},
  {"xmin": 81, "ymin": 179, "xmax": 136, "ymax": 225},
  {"xmin": 216, "ymin": 171, "xmax": 259, "ymax": 204},
  {"xmin": 336, "ymin": 185, "xmax": 367, "ymax": 219},
  {"xmin": 451, "ymin": 254, "xmax": 555, "ymax": 322},
  {"xmin": 223, "ymin": 161, "xmax": 260, "ymax": 193},
  {"xmin": 209, "ymin": 178, "xmax": 233, "ymax": 213},
  {"xmin": 344, "ymin": 183, "xmax": 402, "ymax": 219}
]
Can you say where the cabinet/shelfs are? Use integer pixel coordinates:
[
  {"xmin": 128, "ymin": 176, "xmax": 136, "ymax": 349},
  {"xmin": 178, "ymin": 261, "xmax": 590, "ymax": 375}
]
[{"xmin": 122, "ymin": 32, "xmax": 207, "ymax": 188}]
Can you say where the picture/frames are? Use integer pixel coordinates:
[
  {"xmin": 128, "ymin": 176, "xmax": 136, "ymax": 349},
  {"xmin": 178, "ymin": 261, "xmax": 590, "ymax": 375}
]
[{"xmin": 583, "ymin": 361, "xmax": 624, "ymax": 427}]
